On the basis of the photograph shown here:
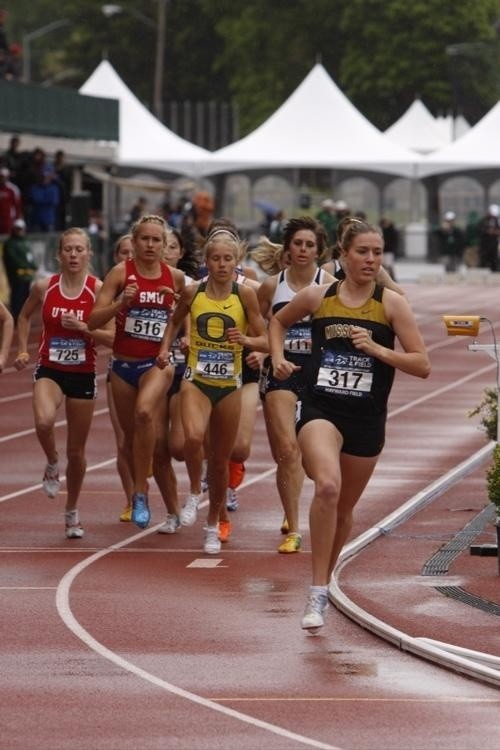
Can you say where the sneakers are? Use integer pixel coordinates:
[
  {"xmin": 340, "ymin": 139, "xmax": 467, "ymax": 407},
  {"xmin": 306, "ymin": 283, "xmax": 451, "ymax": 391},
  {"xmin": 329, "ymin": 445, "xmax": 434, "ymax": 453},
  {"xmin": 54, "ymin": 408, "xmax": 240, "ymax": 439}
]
[
  {"xmin": 302, "ymin": 585, "xmax": 328, "ymax": 630},
  {"xmin": 40, "ymin": 458, "xmax": 304, "ymax": 557}
]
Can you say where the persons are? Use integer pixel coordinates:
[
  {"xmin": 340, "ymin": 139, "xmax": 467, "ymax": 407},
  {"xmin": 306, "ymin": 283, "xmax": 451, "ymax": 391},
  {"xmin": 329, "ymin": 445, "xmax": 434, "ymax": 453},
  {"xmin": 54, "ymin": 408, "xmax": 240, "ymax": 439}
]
[
  {"xmin": 313, "ymin": 197, "xmax": 399, "ymax": 256},
  {"xmin": 132, "ymin": 190, "xmax": 214, "ymax": 230},
  {"xmin": 0, "ymin": 218, "xmax": 38, "ymax": 325},
  {"xmin": 268, "ymin": 215, "xmax": 433, "ymax": 634},
  {"xmin": 431, "ymin": 204, "xmax": 500, "ymax": 272},
  {"xmin": 269, "ymin": 210, "xmax": 291, "ymax": 243},
  {"xmin": 0, "ymin": 215, "xmax": 410, "ymax": 555},
  {"xmin": 0, "ymin": 136, "xmax": 103, "ymax": 233}
]
